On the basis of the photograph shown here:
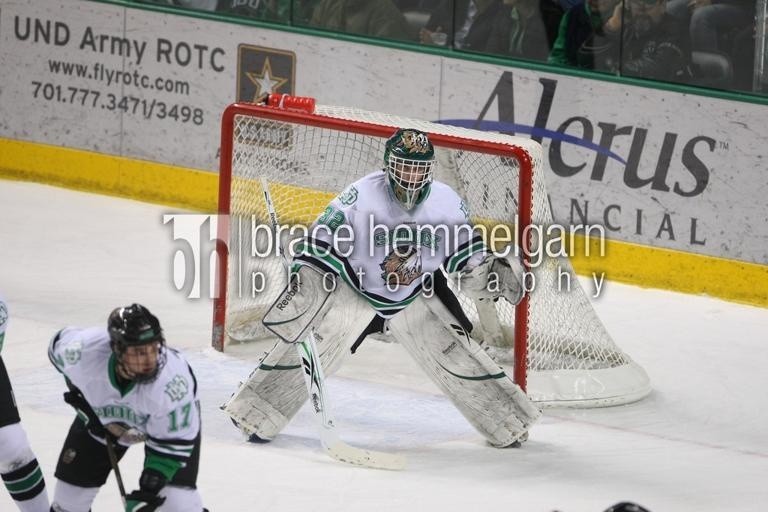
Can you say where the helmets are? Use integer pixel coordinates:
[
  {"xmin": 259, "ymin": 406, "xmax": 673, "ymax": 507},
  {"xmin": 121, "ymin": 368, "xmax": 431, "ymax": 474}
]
[
  {"xmin": 106, "ymin": 303, "xmax": 166, "ymax": 385},
  {"xmin": 383, "ymin": 129, "xmax": 434, "ymax": 191}
]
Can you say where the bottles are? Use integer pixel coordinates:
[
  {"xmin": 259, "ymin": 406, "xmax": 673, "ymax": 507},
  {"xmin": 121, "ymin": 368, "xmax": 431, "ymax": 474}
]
[{"xmin": 262, "ymin": 93, "xmax": 316, "ymax": 114}]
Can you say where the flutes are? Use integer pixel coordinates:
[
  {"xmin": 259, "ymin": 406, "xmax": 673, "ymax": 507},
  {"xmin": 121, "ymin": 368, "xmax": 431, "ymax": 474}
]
[{"xmin": 259, "ymin": 175, "xmax": 402, "ymax": 472}]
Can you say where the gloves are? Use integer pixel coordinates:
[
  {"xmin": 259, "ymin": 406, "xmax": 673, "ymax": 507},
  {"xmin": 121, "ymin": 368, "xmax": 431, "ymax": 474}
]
[
  {"xmin": 124, "ymin": 470, "xmax": 168, "ymax": 512},
  {"xmin": 63, "ymin": 389, "xmax": 118, "ymax": 446}
]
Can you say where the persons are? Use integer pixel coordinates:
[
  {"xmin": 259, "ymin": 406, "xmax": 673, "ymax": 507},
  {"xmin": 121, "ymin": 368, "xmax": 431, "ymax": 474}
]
[
  {"xmin": 0, "ymin": 304, "xmax": 47, "ymax": 511},
  {"xmin": 218, "ymin": 125, "xmax": 542, "ymax": 449},
  {"xmin": 44, "ymin": 304, "xmax": 212, "ymax": 511}
]
